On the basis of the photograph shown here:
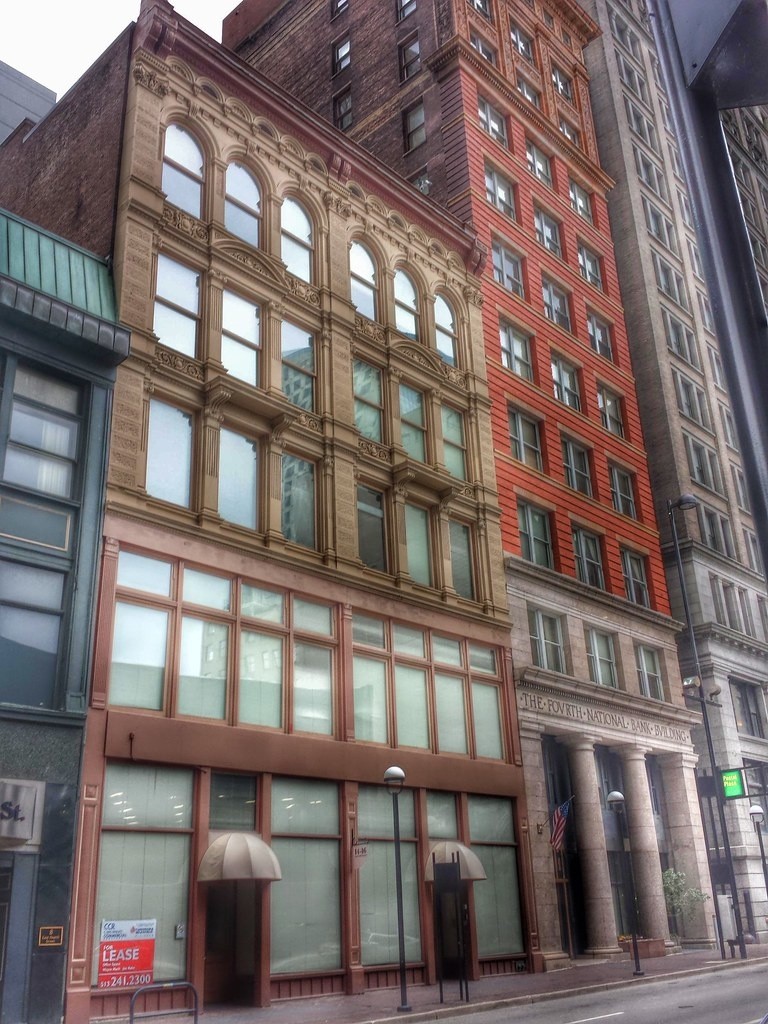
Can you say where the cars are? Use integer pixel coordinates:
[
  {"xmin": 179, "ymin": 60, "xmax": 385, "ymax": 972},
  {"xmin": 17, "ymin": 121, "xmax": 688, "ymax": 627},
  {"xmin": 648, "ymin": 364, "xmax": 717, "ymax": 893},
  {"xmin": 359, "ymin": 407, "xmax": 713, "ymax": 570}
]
[{"xmin": 319, "ymin": 931, "xmax": 416, "ymax": 953}]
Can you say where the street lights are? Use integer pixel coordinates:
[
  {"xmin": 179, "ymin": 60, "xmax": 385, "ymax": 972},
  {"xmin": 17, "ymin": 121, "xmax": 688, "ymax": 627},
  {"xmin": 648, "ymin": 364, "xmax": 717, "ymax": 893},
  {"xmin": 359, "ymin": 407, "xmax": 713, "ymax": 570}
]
[
  {"xmin": 668, "ymin": 493, "xmax": 749, "ymax": 960},
  {"xmin": 751, "ymin": 805, "xmax": 767, "ymax": 894},
  {"xmin": 381, "ymin": 766, "xmax": 411, "ymax": 1012},
  {"xmin": 608, "ymin": 792, "xmax": 645, "ymax": 976}
]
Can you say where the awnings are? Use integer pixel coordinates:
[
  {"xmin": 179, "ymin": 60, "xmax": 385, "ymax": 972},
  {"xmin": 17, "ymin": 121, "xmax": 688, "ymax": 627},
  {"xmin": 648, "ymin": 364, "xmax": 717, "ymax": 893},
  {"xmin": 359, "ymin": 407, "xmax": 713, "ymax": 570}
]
[
  {"xmin": 197, "ymin": 833, "xmax": 282, "ymax": 881},
  {"xmin": 424, "ymin": 842, "xmax": 487, "ymax": 882}
]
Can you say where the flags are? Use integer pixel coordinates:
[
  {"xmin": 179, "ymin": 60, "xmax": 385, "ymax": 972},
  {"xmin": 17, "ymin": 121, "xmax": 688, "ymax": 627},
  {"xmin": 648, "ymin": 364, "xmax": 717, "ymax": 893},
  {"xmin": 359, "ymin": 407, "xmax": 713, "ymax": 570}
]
[{"xmin": 550, "ymin": 800, "xmax": 569, "ymax": 853}]
